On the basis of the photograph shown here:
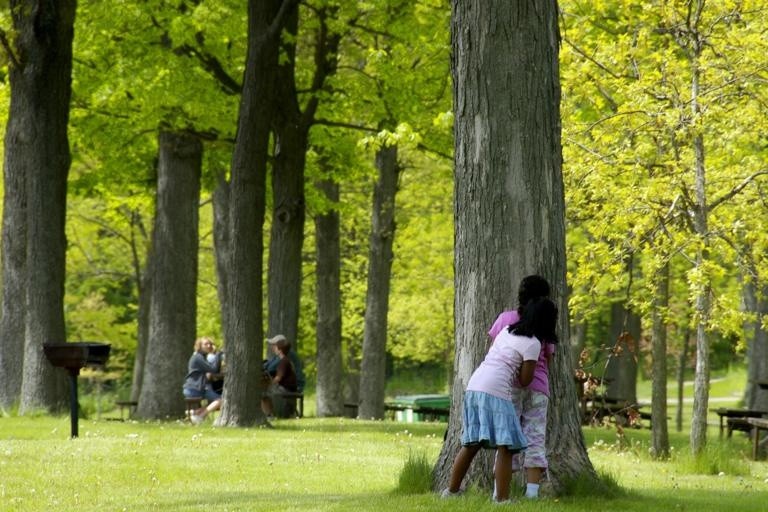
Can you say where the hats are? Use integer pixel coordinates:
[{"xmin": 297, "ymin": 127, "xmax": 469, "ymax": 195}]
[{"xmin": 267, "ymin": 335, "xmax": 285, "ymax": 344}]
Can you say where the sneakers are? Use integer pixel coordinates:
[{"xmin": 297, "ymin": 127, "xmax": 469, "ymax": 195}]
[
  {"xmin": 186, "ymin": 409, "xmax": 201, "ymax": 425},
  {"xmin": 440, "ymin": 488, "xmax": 461, "ymax": 498}
]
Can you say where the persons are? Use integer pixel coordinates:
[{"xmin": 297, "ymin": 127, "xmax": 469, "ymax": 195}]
[
  {"xmin": 441, "ymin": 295, "xmax": 561, "ymax": 506},
  {"xmin": 182, "ymin": 331, "xmax": 306, "ymax": 427},
  {"xmin": 489, "ymin": 272, "xmax": 555, "ymax": 502}
]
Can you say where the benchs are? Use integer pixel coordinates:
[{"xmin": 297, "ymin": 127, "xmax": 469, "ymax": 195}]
[
  {"xmin": 705, "ymin": 404, "xmax": 768, "ymax": 459},
  {"xmin": 345, "ymin": 402, "xmax": 406, "ymax": 421},
  {"xmin": 259, "ymin": 393, "xmax": 304, "ymax": 420},
  {"xmin": 415, "ymin": 406, "xmax": 451, "ymax": 424},
  {"xmin": 114, "ymin": 397, "xmax": 202, "ymax": 421}
]
